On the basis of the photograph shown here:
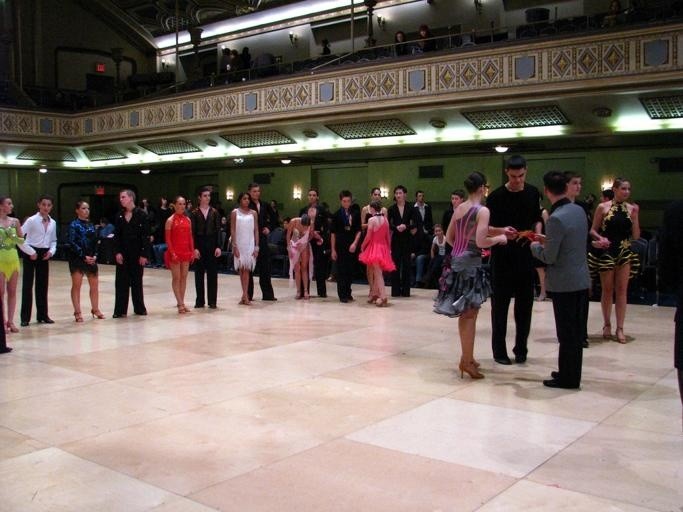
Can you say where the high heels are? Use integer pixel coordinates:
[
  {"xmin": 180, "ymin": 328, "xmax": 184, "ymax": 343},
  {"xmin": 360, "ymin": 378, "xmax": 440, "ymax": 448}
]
[
  {"xmin": 7, "ymin": 320, "xmax": 19, "ymax": 333},
  {"xmin": 376, "ymin": 297, "xmax": 387, "ymax": 306},
  {"xmin": 4, "ymin": 320, "xmax": 9, "ymax": 334},
  {"xmin": 74, "ymin": 312, "xmax": 83, "ymax": 322},
  {"xmin": 458, "ymin": 360, "xmax": 484, "ymax": 380},
  {"xmin": 367, "ymin": 295, "xmax": 377, "ymax": 303},
  {"xmin": 91, "ymin": 309, "xmax": 105, "ymax": 319}
]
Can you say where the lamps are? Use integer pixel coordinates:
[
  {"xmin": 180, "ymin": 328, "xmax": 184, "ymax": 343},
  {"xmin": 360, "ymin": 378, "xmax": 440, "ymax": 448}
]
[
  {"xmin": 226, "ymin": 191, "xmax": 235, "ymax": 202},
  {"xmin": 280, "ymin": 158, "xmax": 292, "ymax": 165},
  {"xmin": 495, "ymin": 143, "xmax": 510, "ymax": 153},
  {"xmin": 38, "ymin": 165, "xmax": 48, "ymax": 175},
  {"xmin": 140, "ymin": 167, "xmax": 152, "ymax": 175},
  {"xmin": 293, "ymin": 185, "xmax": 302, "ymax": 202}
]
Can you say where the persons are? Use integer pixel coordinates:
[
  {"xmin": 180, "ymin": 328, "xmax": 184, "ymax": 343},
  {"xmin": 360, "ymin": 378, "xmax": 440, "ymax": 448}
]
[
  {"xmin": 282, "ymin": 218, "xmax": 292, "ymax": 253},
  {"xmin": 135, "ymin": 200, "xmax": 144, "ymax": 209},
  {"xmin": 287, "ymin": 214, "xmax": 323, "ymax": 300},
  {"xmin": 269, "ymin": 200, "xmax": 279, "ymax": 222},
  {"xmin": 590, "ymin": 177, "xmax": 640, "ymax": 344},
  {"xmin": 582, "ymin": 191, "xmax": 596, "ymax": 205},
  {"xmin": 231, "ymin": 50, "xmax": 240, "ymax": 70},
  {"xmin": 17, "ymin": 194, "xmax": 57, "ymax": 326},
  {"xmin": 168, "ymin": 201, "xmax": 175, "ymax": 210},
  {"xmin": 428, "ymin": 223, "xmax": 448, "ymax": 285},
  {"xmin": 260, "ymin": 224, "xmax": 285, "ymax": 245},
  {"xmin": 190, "ymin": 188, "xmax": 222, "ymax": 309},
  {"xmin": 154, "ymin": 196, "xmax": 172, "ymax": 244},
  {"xmin": 411, "ymin": 190, "xmax": 432, "ymax": 256},
  {"xmin": 432, "ymin": 171, "xmax": 508, "ymax": 380},
  {"xmin": 394, "ymin": 31, "xmax": 407, "ymax": 56},
  {"xmin": 0, "ymin": 196, "xmax": 25, "ymax": 334},
  {"xmin": 564, "ymin": 172, "xmax": 590, "ymax": 348},
  {"xmin": 298, "ymin": 190, "xmax": 329, "ymax": 297},
  {"xmin": 68, "ymin": 200, "xmax": 106, "ymax": 322},
  {"xmin": 240, "ymin": 47, "xmax": 251, "ymax": 69},
  {"xmin": 231, "ymin": 192, "xmax": 260, "ymax": 306},
  {"xmin": 486, "ymin": 155, "xmax": 542, "ymax": 365},
  {"xmin": 599, "ymin": 189, "xmax": 614, "ymax": 202},
  {"xmin": 220, "ymin": 215, "xmax": 230, "ymax": 239},
  {"xmin": 0, "ymin": 298, "xmax": 13, "ymax": 355},
  {"xmin": 387, "ymin": 185, "xmax": 421, "ymax": 297},
  {"xmin": 94, "ymin": 217, "xmax": 115, "ymax": 238},
  {"xmin": 657, "ymin": 199, "xmax": 683, "ymax": 421},
  {"xmin": 419, "ymin": 24, "xmax": 439, "ymax": 51},
  {"xmin": 142, "ymin": 197, "xmax": 160, "ymax": 269},
  {"xmin": 164, "ymin": 197, "xmax": 196, "ymax": 314},
  {"xmin": 330, "ymin": 191, "xmax": 361, "ymax": 303},
  {"xmin": 219, "ymin": 48, "xmax": 233, "ymax": 71},
  {"xmin": 361, "ymin": 187, "xmax": 387, "ymax": 299},
  {"xmin": 358, "ymin": 200, "xmax": 397, "ymax": 307},
  {"xmin": 602, "ymin": 0, "xmax": 626, "ymax": 28},
  {"xmin": 441, "ymin": 188, "xmax": 465, "ymax": 235},
  {"xmin": 186, "ymin": 199, "xmax": 194, "ymax": 209},
  {"xmin": 112, "ymin": 190, "xmax": 150, "ymax": 319},
  {"xmin": 247, "ymin": 183, "xmax": 281, "ymax": 302},
  {"xmin": 530, "ymin": 170, "xmax": 591, "ymax": 388}
]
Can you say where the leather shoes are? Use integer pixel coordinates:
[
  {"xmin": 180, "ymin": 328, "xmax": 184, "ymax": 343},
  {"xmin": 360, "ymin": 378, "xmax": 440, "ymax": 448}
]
[
  {"xmin": 495, "ymin": 357, "xmax": 512, "ymax": 365},
  {"xmin": 136, "ymin": 309, "xmax": 147, "ymax": 316},
  {"xmin": 113, "ymin": 312, "xmax": 124, "ymax": 318},
  {"xmin": 543, "ymin": 377, "xmax": 580, "ymax": 389},
  {"xmin": 262, "ymin": 297, "xmax": 277, "ymax": 300},
  {"xmin": 550, "ymin": 371, "xmax": 561, "ymax": 378},
  {"xmin": 340, "ymin": 297, "xmax": 347, "ymax": 303},
  {"xmin": 514, "ymin": 355, "xmax": 526, "ymax": 364},
  {"xmin": 37, "ymin": 317, "xmax": 54, "ymax": 324},
  {"xmin": 20, "ymin": 320, "xmax": 29, "ymax": 326}
]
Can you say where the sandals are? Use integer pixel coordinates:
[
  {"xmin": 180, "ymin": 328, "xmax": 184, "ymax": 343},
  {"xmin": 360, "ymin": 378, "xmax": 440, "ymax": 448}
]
[
  {"xmin": 183, "ymin": 304, "xmax": 191, "ymax": 312},
  {"xmin": 177, "ymin": 304, "xmax": 185, "ymax": 313},
  {"xmin": 615, "ymin": 326, "xmax": 626, "ymax": 344},
  {"xmin": 304, "ymin": 289, "xmax": 309, "ymax": 300},
  {"xmin": 295, "ymin": 291, "xmax": 301, "ymax": 300},
  {"xmin": 603, "ymin": 324, "xmax": 611, "ymax": 338}
]
